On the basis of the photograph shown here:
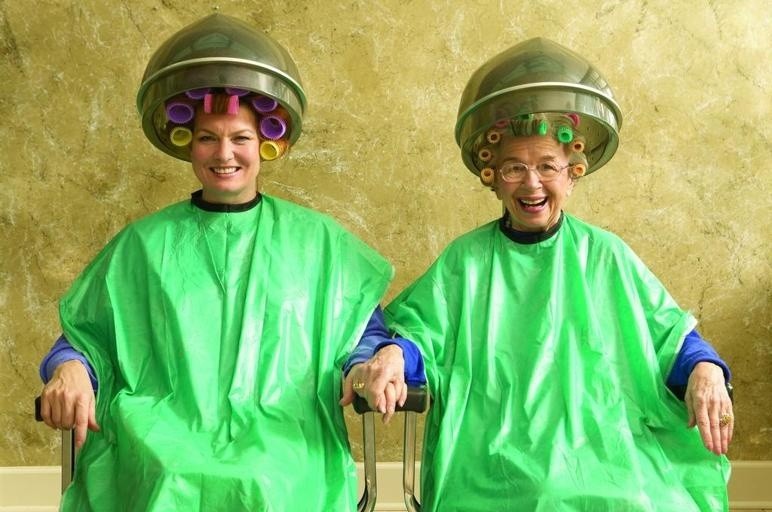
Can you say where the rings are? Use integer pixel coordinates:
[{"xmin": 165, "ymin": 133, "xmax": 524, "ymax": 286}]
[
  {"xmin": 720, "ymin": 415, "xmax": 732, "ymax": 428},
  {"xmin": 352, "ymin": 383, "xmax": 365, "ymax": 389}
]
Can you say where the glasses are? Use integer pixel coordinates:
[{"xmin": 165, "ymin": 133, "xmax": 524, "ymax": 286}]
[{"xmin": 499, "ymin": 160, "xmax": 575, "ymax": 183}]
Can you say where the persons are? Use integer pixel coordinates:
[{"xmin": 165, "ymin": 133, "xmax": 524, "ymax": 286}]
[
  {"xmin": 352, "ymin": 113, "xmax": 736, "ymax": 512},
  {"xmin": 39, "ymin": 83, "xmax": 408, "ymax": 512}
]
